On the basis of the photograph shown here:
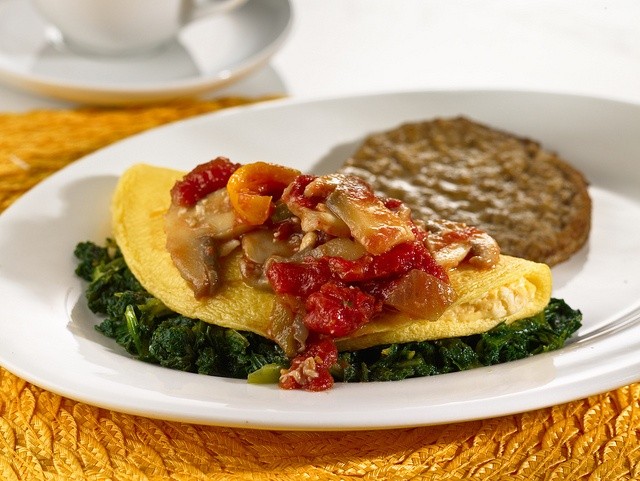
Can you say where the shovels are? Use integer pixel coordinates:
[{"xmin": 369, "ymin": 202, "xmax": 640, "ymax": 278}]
[
  {"xmin": 115, "ymin": 162, "xmax": 554, "ymax": 353},
  {"xmin": 341, "ymin": 116, "xmax": 591, "ymax": 269}
]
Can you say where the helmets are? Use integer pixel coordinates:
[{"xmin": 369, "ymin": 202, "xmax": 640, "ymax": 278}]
[
  {"xmin": 0, "ymin": 93, "xmax": 640, "ymax": 434},
  {"xmin": 0, "ymin": 2, "xmax": 296, "ymax": 109}
]
